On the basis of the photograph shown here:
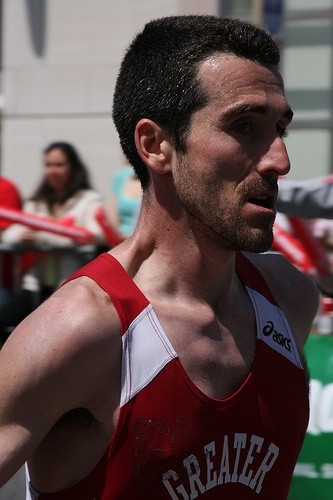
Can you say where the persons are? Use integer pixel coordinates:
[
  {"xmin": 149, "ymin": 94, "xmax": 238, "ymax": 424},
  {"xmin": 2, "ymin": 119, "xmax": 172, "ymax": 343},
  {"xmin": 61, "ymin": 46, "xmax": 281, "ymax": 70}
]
[
  {"xmin": 0, "ymin": 141, "xmax": 148, "ymax": 314},
  {"xmin": 0, "ymin": 15, "xmax": 320, "ymax": 500}
]
